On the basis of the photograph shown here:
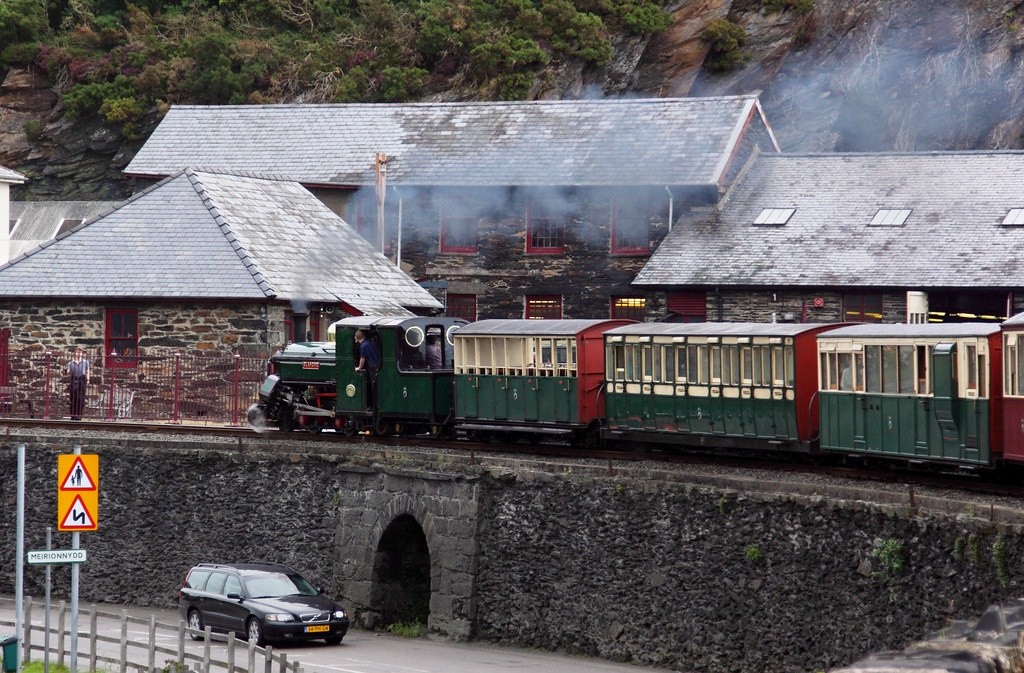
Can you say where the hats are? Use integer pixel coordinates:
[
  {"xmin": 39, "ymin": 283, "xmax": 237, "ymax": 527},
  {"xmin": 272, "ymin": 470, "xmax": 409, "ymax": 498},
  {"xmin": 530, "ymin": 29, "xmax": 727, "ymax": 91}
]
[{"xmin": 426, "ymin": 335, "xmax": 439, "ymax": 342}]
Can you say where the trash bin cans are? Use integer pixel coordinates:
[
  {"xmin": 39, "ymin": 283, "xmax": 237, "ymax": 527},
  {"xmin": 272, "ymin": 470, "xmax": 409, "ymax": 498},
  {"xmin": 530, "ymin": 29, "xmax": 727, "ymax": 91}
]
[{"xmin": 0, "ymin": 635, "xmax": 17, "ymax": 672}]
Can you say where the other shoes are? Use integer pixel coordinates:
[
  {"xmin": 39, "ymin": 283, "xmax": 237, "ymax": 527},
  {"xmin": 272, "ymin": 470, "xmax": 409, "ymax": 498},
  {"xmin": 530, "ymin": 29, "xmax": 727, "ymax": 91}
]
[
  {"xmin": 365, "ymin": 408, "xmax": 374, "ymax": 413},
  {"xmin": 70, "ymin": 418, "xmax": 81, "ymax": 420}
]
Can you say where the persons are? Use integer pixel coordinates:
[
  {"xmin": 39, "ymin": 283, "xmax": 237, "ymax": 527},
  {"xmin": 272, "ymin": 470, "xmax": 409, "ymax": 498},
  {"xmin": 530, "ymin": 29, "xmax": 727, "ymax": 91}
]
[
  {"xmin": 426, "ymin": 335, "xmax": 442, "ymax": 369},
  {"xmin": 63, "ymin": 347, "xmax": 90, "ymax": 419},
  {"xmin": 354, "ymin": 329, "xmax": 381, "ymax": 411}
]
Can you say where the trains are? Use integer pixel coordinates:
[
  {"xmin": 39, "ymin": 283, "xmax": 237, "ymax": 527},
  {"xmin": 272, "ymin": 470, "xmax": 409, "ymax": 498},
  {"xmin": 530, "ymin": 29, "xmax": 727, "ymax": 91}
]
[{"xmin": 247, "ymin": 315, "xmax": 1024, "ymax": 482}]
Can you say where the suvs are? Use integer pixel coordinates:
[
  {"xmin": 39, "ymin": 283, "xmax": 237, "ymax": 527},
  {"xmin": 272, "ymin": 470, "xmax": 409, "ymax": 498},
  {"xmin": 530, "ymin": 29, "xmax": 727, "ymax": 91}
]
[{"xmin": 178, "ymin": 561, "xmax": 349, "ymax": 647}]
